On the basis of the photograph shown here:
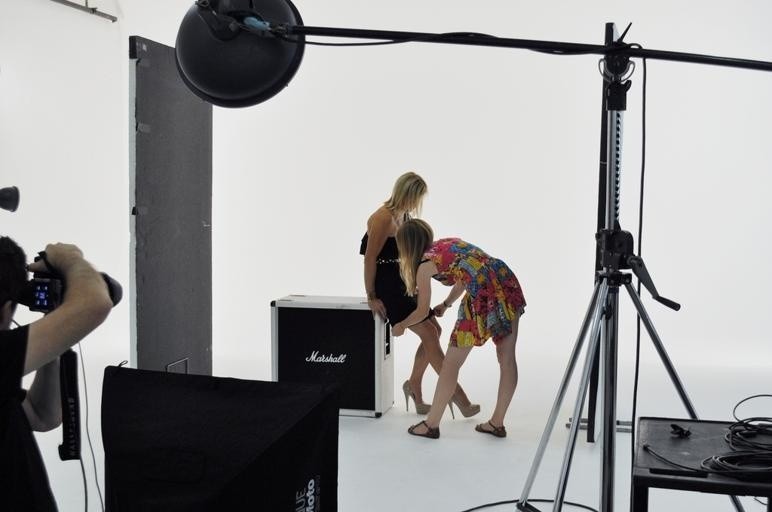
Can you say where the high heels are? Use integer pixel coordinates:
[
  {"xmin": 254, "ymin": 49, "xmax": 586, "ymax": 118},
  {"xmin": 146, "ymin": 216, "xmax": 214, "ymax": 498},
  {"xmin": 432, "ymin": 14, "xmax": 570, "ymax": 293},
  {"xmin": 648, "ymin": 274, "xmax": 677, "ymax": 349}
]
[
  {"xmin": 403, "ymin": 381, "xmax": 432, "ymax": 415},
  {"xmin": 448, "ymin": 393, "xmax": 481, "ymax": 420}
]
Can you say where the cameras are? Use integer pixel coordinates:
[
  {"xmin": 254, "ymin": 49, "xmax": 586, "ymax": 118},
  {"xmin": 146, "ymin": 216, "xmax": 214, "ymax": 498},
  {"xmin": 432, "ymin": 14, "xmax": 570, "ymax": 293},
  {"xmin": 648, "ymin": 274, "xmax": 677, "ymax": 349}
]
[{"xmin": 19, "ymin": 250, "xmax": 123, "ymax": 316}]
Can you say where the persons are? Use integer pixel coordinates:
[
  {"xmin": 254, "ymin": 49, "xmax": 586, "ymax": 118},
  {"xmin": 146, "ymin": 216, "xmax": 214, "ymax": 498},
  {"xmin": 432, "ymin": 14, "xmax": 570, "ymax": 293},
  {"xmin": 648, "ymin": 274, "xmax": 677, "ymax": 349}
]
[
  {"xmin": 387, "ymin": 218, "xmax": 527, "ymax": 441},
  {"xmin": 0, "ymin": 233, "xmax": 114, "ymax": 512},
  {"xmin": 364, "ymin": 171, "xmax": 480, "ymax": 422}
]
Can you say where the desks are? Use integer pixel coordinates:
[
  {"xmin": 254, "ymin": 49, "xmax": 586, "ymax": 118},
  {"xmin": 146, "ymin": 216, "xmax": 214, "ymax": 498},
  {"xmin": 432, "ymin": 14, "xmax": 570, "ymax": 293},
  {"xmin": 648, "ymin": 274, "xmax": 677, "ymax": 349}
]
[{"xmin": 630, "ymin": 417, "xmax": 772, "ymax": 512}]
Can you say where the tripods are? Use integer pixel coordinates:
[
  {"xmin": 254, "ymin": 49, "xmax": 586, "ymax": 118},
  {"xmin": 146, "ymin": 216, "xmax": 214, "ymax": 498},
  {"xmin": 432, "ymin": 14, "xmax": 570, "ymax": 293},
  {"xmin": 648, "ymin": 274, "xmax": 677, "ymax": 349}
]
[{"xmin": 517, "ymin": 75, "xmax": 743, "ymax": 512}]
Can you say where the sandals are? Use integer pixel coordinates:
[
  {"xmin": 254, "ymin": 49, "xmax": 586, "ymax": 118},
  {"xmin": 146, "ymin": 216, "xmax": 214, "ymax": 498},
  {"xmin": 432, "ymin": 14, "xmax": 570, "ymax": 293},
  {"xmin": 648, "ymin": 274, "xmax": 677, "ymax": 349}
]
[
  {"xmin": 409, "ymin": 421, "xmax": 442, "ymax": 440},
  {"xmin": 476, "ymin": 421, "xmax": 507, "ymax": 439}
]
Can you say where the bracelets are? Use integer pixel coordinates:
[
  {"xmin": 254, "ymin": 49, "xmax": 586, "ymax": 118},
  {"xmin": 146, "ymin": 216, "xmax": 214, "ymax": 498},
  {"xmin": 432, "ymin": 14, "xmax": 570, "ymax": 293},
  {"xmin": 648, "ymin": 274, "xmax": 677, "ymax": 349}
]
[{"xmin": 444, "ymin": 302, "xmax": 451, "ymax": 307}]
